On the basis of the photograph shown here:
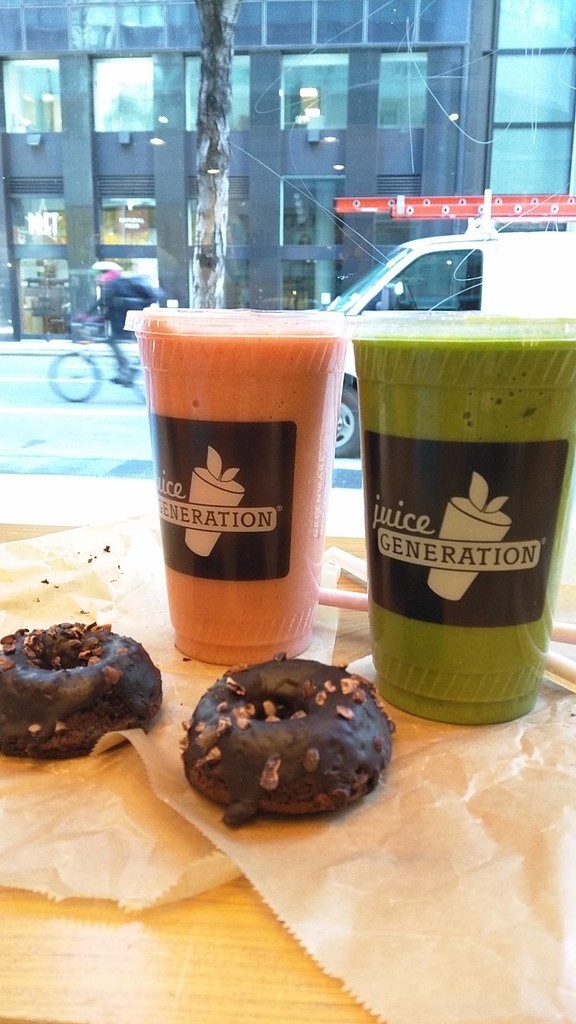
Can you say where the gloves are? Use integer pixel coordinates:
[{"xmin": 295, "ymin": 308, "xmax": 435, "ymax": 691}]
[
  {"xmin": 71, "ymin": 314, "xmax": 88, "ymax": 321},
  {"xmin": 89, "ymin": 316, "xmax": 105, "ymax": 321}
]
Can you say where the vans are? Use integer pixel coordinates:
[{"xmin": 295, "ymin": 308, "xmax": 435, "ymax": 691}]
[{"xmin": 323, "ymin": 227, "xmax": 575, "ymax": 458}]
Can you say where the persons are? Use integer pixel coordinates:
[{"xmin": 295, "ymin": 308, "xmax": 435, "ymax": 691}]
[{"xmin": 69, "ymin": 260, "xmax": 168, "ymax": 387}]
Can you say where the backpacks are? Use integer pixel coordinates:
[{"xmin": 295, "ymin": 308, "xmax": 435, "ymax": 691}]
[{"xmin": 113, "ymin": 271, "xmax": 166, "ymax": 302}]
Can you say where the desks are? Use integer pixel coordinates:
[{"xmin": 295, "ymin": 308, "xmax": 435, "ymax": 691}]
[
  {"xmin": 28, "ymin": 277, "xmax": 68, "ymax": 336},
  {"xmin": 0, "ymin": 525, "xmax": 576, "ymax": 1024}
]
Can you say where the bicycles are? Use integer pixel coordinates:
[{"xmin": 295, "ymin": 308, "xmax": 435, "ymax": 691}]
[{"xmin": 47, "ymin": 316, "xmax": 145, "ymax": 407}]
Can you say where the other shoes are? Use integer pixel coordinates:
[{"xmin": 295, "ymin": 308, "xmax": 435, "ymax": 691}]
[{"xmin": 111, "ymin": 366, "xmax": 138, "ymax": 386}]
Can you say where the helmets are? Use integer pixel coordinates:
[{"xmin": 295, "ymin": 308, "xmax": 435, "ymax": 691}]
[{"xmin": 91, "ymin": 261, "xmax": 123, "ymax": 271}]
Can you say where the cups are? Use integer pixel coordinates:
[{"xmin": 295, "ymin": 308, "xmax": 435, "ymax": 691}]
[
  {"xmin": 347, "ymin": 313, "xmax": 576, "ymax": 723},
  {"xmin": 124, "ymin": 308, "xmax": 352, "ymax": 668}
]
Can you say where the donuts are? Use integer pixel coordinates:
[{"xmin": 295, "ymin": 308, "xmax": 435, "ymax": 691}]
[
  {"xmin": 0, "ymin": 621, "xmax": 163, "ymax": 758},
  {"xmin": 179, "ymin": 651, "xmax": 398, "ymax": 826}
]
